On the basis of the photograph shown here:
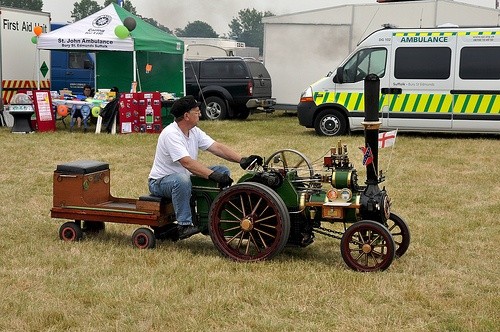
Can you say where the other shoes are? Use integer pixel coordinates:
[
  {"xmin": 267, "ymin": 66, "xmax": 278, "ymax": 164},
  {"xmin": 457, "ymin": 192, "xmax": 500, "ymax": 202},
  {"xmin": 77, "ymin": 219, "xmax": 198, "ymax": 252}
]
[{"xmin": 178, "ymin": 225, "xmax": 205, "ymax": 240}]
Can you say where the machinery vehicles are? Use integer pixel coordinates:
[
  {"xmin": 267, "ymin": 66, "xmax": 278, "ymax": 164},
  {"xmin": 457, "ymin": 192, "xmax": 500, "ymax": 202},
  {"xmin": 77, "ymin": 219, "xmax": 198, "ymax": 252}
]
[{"xmin": 50, "ymin": 73, "xmax": 409, "ymax": 276}]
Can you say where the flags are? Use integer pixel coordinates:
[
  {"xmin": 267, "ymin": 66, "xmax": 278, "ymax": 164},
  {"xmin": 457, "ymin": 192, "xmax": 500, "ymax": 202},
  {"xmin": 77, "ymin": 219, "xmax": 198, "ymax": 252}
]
[
  {"xmin": 378, "ymin": 130, "xmax": 397, "ymax": 148},
  {"xmin": 358, "ymin": 146, "xmax": 373, "ymax": 166}
]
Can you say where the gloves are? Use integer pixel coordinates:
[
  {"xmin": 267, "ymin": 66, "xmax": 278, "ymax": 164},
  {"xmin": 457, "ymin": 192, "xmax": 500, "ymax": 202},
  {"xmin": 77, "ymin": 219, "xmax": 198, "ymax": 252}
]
[
  {"xmin": 208, "ymin": 171, "xmax": 234, "ymax": 189},
  {"xmin": 240, "ymin": 155, "xmax": 263, "ymax": 170}
]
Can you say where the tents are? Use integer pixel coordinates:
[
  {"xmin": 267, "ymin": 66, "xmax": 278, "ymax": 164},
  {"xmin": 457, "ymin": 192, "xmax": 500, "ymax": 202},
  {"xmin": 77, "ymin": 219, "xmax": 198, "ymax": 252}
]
[{"xmin": 36, "ymin": 2, "xmax": 186, "ymax": 96}]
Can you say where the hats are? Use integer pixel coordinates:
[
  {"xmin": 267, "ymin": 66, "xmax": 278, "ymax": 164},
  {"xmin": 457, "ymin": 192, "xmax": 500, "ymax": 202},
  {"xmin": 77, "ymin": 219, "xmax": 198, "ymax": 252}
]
[
  {"xmin": 111, "ymin": 87, "xmax": 119, "ymax": 92},
  {"xmin": 170, "ymin": 95, "xmax": 202, "ymax": 118}
]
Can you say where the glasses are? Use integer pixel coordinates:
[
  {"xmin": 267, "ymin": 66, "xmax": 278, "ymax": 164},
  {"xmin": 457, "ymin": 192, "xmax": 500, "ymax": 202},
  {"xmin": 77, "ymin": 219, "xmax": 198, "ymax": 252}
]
[{"xmin": 189, "ymin": 110, "xmax": 201, "ymax": 115}]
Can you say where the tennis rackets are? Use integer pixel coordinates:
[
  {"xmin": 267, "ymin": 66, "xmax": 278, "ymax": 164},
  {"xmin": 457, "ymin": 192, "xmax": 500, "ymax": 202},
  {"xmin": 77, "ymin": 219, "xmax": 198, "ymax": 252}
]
[
  {"xmin": 80, "ymin": 105, "xmax": 91, "ymax": 133},
  {"xmin": 70, "ymin": 104, "xmax": 76, "ymax": 132}
]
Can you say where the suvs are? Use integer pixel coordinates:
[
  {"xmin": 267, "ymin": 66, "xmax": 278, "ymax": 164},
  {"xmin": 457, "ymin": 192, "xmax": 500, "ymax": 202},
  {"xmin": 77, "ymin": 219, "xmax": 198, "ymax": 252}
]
[{"xmin": 185, "ymin": 55, "xmax": 277, "ymax": 120}]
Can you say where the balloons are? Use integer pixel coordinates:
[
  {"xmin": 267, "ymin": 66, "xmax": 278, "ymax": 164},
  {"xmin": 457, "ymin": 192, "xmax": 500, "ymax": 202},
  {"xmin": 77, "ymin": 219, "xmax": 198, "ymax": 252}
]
[
  {"xmin": 115, "ymin": 25, "xmax": 130, "ymax": 38},
  {"xmin": 34, "ymin": 26, "xmax": 42, "ymax": 35},
  {"xmin": 32, "ymin": 36, "xmax": 37, "ymax": 44},
  {"xmin": 123, "ymin": 17, "xmax": 136, "ymax": 31}
]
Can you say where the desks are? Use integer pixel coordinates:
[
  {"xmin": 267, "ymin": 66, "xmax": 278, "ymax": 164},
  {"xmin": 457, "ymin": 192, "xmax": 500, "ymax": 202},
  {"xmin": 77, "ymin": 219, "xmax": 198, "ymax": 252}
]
[
  {"xmin": 52, "ymin": 99, "xmax": 116, "ymax": 135},
  {"xmin": 161, "ymin": 97, "xmax": 181, "ymax": 123}
]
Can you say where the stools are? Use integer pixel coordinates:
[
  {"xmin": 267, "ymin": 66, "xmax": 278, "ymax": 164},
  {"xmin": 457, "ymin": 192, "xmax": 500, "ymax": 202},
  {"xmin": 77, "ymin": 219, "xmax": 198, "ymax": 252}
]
[
  {"xmin": 54, "ymin": 158, "xmax": 109, "ymax": 206},
  {"xmin": 138, "ymin": 194, "xmax": 175, "ymax": 212}
]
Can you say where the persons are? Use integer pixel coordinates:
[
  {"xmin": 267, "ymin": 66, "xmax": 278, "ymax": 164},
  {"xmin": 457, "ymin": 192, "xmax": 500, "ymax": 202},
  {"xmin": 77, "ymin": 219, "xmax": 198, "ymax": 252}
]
[
  {"xmin": 148, "ymin": 96, "xmax": 263, "ymax": 239},
  {"xmin": 73, "ymin": 85, "xmax": 95, "ymax": 129},
  {"xmin": 111, "ymin": 87, "xmax": 119, "ymax": 94}
]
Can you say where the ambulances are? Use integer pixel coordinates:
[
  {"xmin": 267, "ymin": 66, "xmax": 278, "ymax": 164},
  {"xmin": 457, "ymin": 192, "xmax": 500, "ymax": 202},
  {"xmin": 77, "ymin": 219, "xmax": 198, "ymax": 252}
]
[{"xmin": 296, "ymin": 21, "xmax": 497, "ymax": 138}]
